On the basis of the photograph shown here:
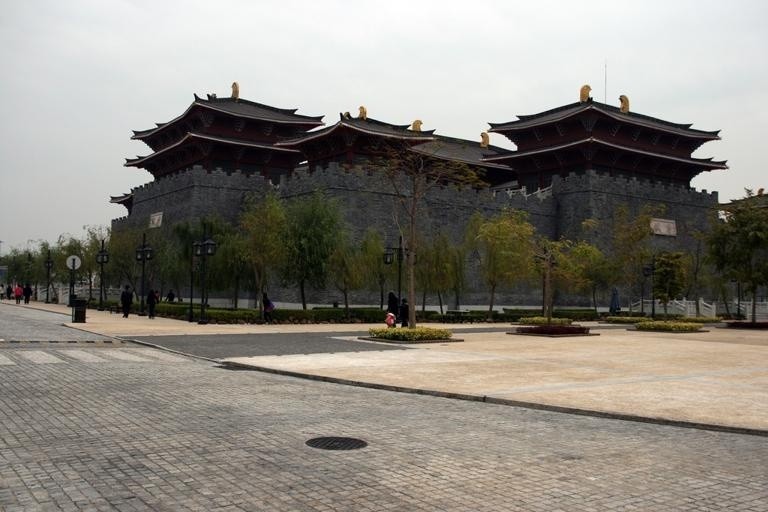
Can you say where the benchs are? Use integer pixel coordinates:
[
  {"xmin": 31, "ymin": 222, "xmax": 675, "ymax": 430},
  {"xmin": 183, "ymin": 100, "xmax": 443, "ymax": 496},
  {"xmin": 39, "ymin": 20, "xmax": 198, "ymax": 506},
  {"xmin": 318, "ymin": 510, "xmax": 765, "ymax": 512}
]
[{"xmin": 108, "ymin": 303, "xmax": 133, "ymax": 314}]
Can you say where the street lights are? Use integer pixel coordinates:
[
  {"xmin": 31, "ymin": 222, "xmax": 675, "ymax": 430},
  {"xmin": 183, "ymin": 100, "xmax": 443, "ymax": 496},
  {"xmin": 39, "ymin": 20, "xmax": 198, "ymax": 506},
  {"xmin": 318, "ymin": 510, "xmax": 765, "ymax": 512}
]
[
  {"xmin": 12, "ymin": 249, "xmax": 53, "ymax": 304},
  {"xmin": 534, "ymin": 245, "xmax": 558, "ymax": 317},
  {"xmin": 67, "ymin": 249, "xmax": 79, "ymax": 307},
  {"xmin": 193, "ymin": 221, "xmax": 215, "ymax": 326},
  {"xmin": 643, "ymin": 255, "xmax": 663, "ymax": 317},
  {"xmin": 95, "ymin": 239, "xmax": 109, "ymax": 311},
  {"xmin": 135, "ymin": 233, "xmax": 153, "ymax": 316},
  {"xmin": 382, "ymin": 235, "xmax": 418, "ymax": 323}
]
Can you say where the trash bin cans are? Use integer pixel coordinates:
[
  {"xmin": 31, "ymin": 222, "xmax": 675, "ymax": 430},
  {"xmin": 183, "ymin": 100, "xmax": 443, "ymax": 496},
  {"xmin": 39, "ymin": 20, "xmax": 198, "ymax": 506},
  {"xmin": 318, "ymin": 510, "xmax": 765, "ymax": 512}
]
[{"xmin": 71, "ymin": 299, "xmax": 87, "ymax": 323}]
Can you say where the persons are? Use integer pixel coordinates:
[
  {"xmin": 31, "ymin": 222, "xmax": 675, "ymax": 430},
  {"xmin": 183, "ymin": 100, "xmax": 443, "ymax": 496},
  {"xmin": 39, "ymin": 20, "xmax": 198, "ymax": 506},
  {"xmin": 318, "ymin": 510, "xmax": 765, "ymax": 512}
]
[
  {"xmin": 1, "ymin": 281, "xmax": 180, "ymax": 320},
  {"xmin": 400, "ymin": 298, "xmax": 411, "ymax": 328},
  {"xmin": 385, "ymin": 291, "xmax": 400, "ymax": 326},
  {"xmin": 261, "ymin": 292, "xmax": 274, "ymax": 325}
]
